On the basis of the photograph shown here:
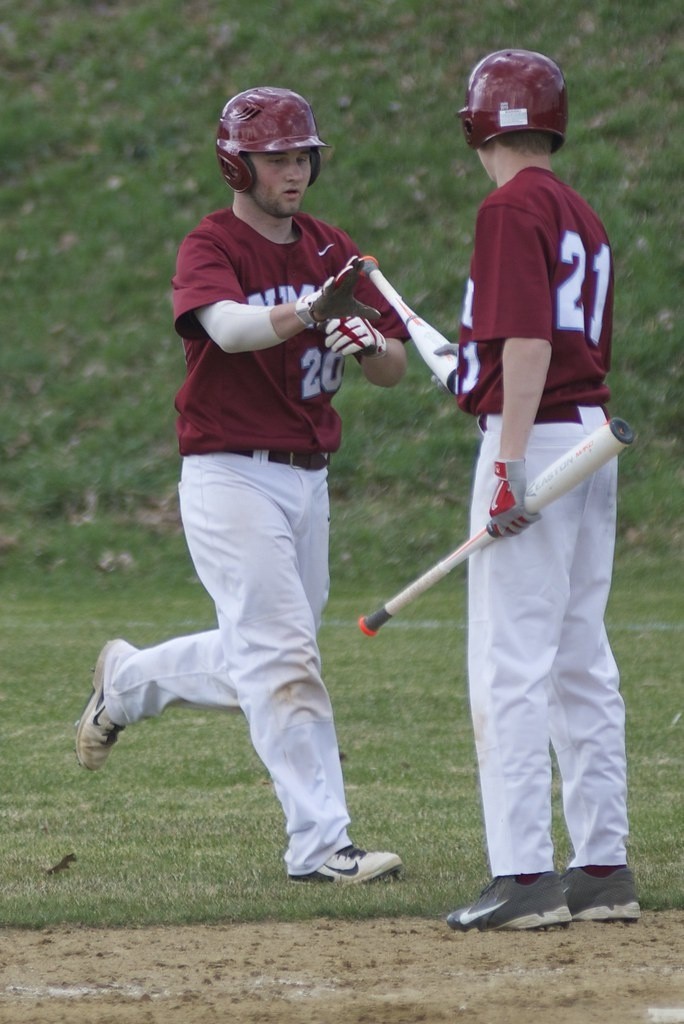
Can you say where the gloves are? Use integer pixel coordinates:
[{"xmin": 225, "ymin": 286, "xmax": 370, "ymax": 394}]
[
  {"xmin": 432, "ymin": 343, "xmax": 470, "ymax": 398},
  {"xmin": 485, "ymin": 457, "xmax": 543, "ymax": 538},
  {"xmin": 297, "ymin": 254, "xmax": 381, "ymax": 322},
  {"xmin": 329, "ymin": 313, "xmax": 388, "ymax": 361}
]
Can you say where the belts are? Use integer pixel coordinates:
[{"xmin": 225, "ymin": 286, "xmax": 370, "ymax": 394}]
[
  {"xmin": 231, "ymin": 449, "xmax": 332, "ymax": 471},
  {"xmin": 478, "ymin": 401, "xmax": 611, "ymax": 433}
]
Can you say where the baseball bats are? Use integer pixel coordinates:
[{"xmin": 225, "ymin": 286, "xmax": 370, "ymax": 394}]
[
  {"xmin": 357, "ymin": 416, "xmax": 635, "ymax": 636},
  {"xmin": 358, "ymin": 254, "xmax": 459, "ymax": 397}
]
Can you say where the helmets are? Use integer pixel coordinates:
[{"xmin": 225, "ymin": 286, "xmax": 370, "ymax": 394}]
[
  {"xmin": 215, "ymin": 87, "xmax": 333, "ymax": 193},
  {"xmin": 456, "ymin": 47, "xmax": 569, "ymax": 156}
]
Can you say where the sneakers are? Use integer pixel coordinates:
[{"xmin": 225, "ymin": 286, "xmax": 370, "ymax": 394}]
[
  {"xmin": 288, "ymin": 845, "xmax": 401, "ymax": 888},
  {"xmin": 563, "ymin": 868, "xmax": 643, "ymax": 921},
  {"xmin": 75, "ymin": 638, "xmax": 127, "ymax": 773},
  {"xmin": 446, "ymin": 871, "xmax": 573, "ymax": 931}
]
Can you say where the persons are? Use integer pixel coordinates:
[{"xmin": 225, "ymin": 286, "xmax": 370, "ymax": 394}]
[
  {"xmin": 430, "ymin": 48, "xmax": 641, "ymax": 931},
  {"xmin": 75, "ymin": 86, "xmax": 412, "ymax": 887}
]
